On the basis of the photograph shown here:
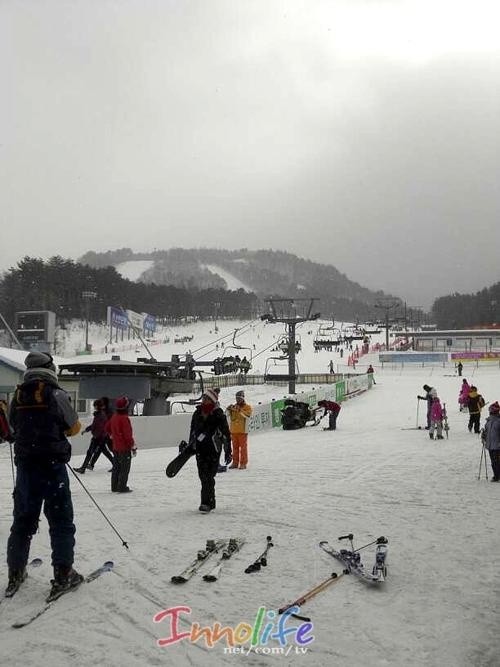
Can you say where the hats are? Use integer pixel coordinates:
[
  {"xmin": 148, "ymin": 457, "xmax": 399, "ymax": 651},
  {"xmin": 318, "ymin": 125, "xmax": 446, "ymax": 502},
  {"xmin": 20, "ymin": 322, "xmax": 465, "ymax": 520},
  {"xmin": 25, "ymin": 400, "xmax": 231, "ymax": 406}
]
[
  {"xmin": 114, "ymin": 396, "xmax": 129, "ymax": 410},
  {"xmin": 23, "ymin": 349, "xmax": 56, "ymax": 372},
  {"xmin": 236, "ymin": 390, "xmax": 244, "ymax": 399},
  {"xmin": 203, "ymin": 387, "xmax": 221, "ymax": 404},
  {"xmin": 488, "ymin": 401, "xmax": 500, "ymax": 412}
]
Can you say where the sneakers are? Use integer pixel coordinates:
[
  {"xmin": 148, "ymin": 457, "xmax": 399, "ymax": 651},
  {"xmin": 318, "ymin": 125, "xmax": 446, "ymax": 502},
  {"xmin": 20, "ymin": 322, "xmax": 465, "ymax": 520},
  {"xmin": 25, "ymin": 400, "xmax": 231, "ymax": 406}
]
[
  {"xmin": 229, "ymin": 463, "xmax": 238, "ymax": 468},
  {"xmin": 198, "ymin": 499, "xmax": 217, "ymax": 514},
  {"xmin": 54, "ymin": 567, "xmax": 85, "ymax": 592},
  {"xmin": 239, "ymin": 464, "xmax": 246, "ymax": 470}
]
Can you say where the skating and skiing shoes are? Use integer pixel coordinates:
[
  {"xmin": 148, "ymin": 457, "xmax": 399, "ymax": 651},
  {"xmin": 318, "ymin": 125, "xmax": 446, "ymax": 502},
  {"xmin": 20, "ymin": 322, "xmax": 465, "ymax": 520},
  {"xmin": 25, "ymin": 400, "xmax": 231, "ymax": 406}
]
[{"xmin": 8, "ymin": 565, "xmax": 28, "ymax": 583}]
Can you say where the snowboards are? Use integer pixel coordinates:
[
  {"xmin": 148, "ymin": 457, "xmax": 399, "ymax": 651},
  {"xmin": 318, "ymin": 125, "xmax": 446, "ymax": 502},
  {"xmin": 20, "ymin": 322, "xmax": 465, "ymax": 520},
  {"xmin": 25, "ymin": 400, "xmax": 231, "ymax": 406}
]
[{"xmin": 166, "ymin": 437, "xmax": 198, "ymax": 478}]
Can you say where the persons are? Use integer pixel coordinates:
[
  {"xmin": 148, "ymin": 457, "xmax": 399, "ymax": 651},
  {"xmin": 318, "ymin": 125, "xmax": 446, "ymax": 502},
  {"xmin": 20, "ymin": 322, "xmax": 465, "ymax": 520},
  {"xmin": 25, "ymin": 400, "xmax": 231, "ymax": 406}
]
[
  {"xmin": 166, "ymin": 387, "xmax": 232, "ymax": 514},
  {"xmin": 85, "ymin": 396, "xmax": 113, "ymax": 470},
  {"xmin": 328, "ymin": 360, "xmax": 334, "ymax": 374},
  {"xmin": 468, "ymin": 386, "xmax": 485, "ymax": 433},
  {"xmin": 0, "ymin": 399, "xmax": 14, "ymax": 444},
  {"xmin": 429, "ymin": 397, "xmax": 445, "ymax": 440},
  {"xmin": 73, "ymin": 400, "xmax": 115, "ymax": 474},
  {"xmin": 335, "ymin": 321, "xmax": 384, "ymax": 359},
  {"xmin": 3, "ymin": 351, "xmax": 84, "ymax": 600},
  {"xmin": 313, "ymin": 339, "xmax": 333, "ymax": 352},
  {"xmin": 481, "ymin": 401, "xmax": 500, "ymax": 482},
  {"xmin": 306, "ymin": 328, "xmax": 313, "ymax": 335},
  {"xmin": 104, "ymin": 396, "xmax": 138, "ymax": 493},
  {"xmin": 318, "ymin": 400, "xmax": 341, "ymax": 430},
  {"xmin": 279, "ymin": 340, "xmax": 300, "ymax": 355},
  {"xmin": 159, "ymin": 320, "xmax": 276, "ymax": 376},
  {"xmin": 366, "ymin": 364, "xmax": 376, "ymax": 384},
  {"xmin": 418, "ymin": 385, "xmax": 437, "ymax": 430},
  {"xmin": 457, "ymin": 361, "xmax": 463, "ymax": 376},
  {"xmin": 228, "ymin": 390, "xmax": 253, "ymax": 471},
  {"xmin": 458, "ymin": 378, "xmax": 471, "ymax": 410}
]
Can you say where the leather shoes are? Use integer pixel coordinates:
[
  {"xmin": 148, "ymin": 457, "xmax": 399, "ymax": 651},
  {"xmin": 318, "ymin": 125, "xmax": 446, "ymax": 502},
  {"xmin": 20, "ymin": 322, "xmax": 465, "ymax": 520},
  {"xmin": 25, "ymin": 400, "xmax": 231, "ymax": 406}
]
[
  {"xmin": 86, "ymin": 465, "xmax": 93, "ymax": 470},
  {"xmin": 73, "ymin": 467, "xmax": 86, "ymax": 474}
]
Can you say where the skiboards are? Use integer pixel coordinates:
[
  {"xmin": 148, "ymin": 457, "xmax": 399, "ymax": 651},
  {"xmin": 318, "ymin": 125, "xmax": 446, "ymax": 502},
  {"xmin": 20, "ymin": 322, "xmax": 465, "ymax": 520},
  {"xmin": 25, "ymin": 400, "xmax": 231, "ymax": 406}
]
[
  {"xmin": 319, "ymin": 536, "xmax": 388, "ymax": 583},
  {"xmin": 0, "ymin": 559, "xmax": 113, "ymax": 629},
  {"xmin": 402, "ymin": 427, "xmax": 440, "ymax": 430},
  {"xmin": 172, "ymin": 538, "xmax": 244, "ymax": 582}
]
